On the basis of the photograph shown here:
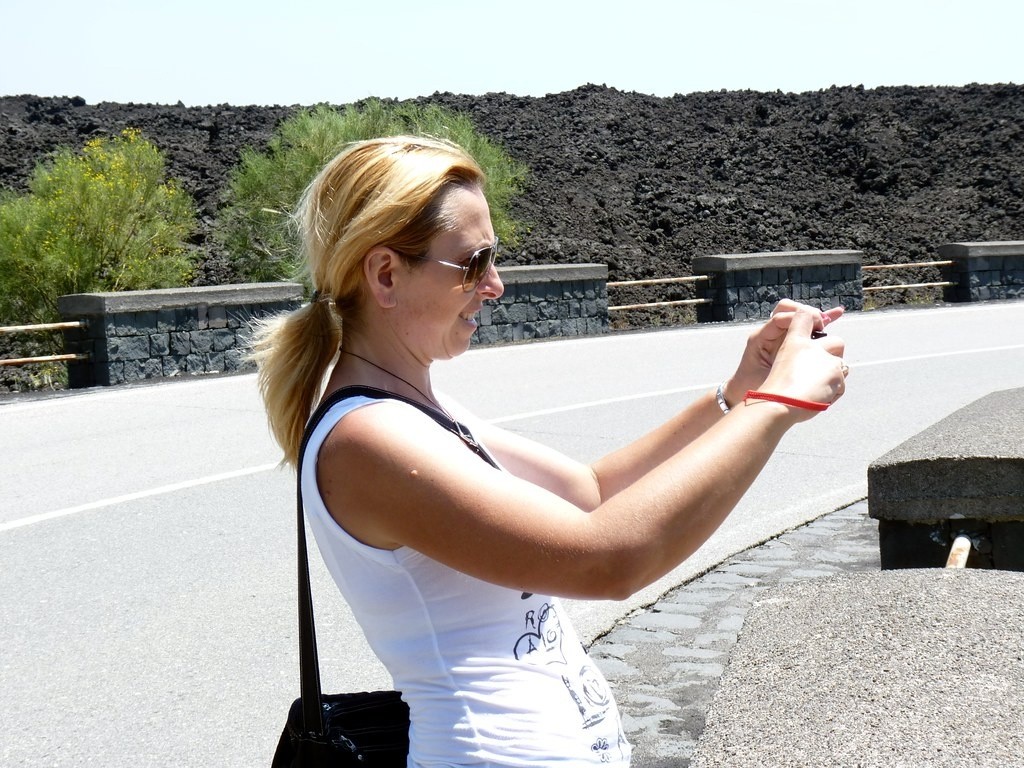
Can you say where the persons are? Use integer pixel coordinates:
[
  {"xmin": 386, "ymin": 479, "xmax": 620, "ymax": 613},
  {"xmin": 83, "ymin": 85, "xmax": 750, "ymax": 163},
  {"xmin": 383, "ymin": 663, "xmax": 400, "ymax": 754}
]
[{"xmin": 241, "ymin": 132, "xmax": 849, "ymax": 767}]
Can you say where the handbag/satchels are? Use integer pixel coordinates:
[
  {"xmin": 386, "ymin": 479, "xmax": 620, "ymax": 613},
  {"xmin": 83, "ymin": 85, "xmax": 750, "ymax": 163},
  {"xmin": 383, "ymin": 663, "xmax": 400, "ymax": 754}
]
[{"xmin": 271, "ymin": 690, "xmax": 411, "ymax": 768}]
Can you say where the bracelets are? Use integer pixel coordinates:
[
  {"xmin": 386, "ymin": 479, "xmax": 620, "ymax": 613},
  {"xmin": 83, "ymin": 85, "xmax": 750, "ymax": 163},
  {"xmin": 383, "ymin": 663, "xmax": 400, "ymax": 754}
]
[{"xmin": 714, "ymin": 371, "xmax": 732, "ymax": 415}]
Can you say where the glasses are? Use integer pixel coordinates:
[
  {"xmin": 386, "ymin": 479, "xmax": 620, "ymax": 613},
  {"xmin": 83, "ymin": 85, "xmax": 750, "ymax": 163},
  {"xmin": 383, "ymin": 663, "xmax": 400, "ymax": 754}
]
[{"xmin": 384, "ymin": 236, "xmax": 499, "ymax": 293}]
